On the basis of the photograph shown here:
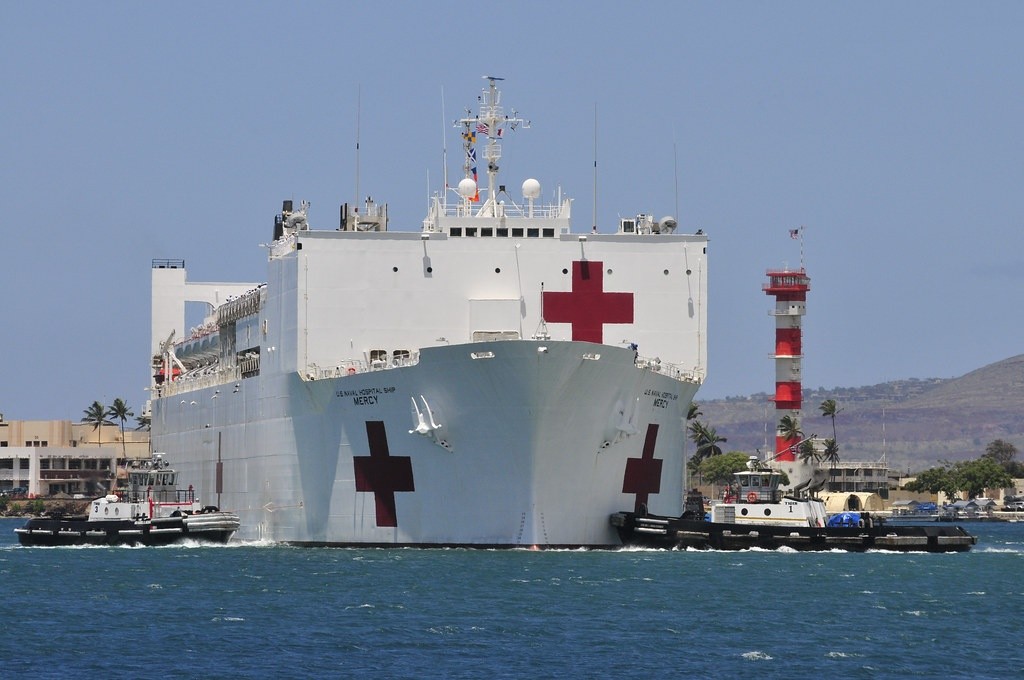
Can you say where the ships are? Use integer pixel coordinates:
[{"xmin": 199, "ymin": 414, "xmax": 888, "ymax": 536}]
[{"xmin": 146, "ymin": 75, "xmax": 710, "ymax": 554}]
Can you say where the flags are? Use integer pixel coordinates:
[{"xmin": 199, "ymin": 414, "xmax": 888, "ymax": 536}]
[
  {"xmin": 497, "ymin": 127, "xmax": 505, "ymax": 137},
  {"xmin": 461, "ymin": 130, "xmax": 475, "ymax": 143},
  {"xmin": 468, "ymin": 188, "xmax": 480, "ymax": 202},
  {"xmin": 476, "ymin": 123, "xmax": 490, "ymax": 134},
  {"xmin": 466, "ymin": 147, "xmax": 478, "ymax": 163},
  {"xmin": 472, "ymin": 167, "xmax": 477, "ymax": 183}
]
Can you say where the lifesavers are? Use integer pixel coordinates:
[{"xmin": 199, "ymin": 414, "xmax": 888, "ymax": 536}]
[{"xmin": 746, "ymin": 492, "xmax": 757, "ymax": 502}]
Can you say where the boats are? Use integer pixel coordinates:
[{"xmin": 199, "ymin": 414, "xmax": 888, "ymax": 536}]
[
  {"xmin": 153, "ymin": 367, "xmax": 180, "ymax": 384},
  {"xmin": 708, "ymin": 431, "xmax": 826, "ymax": 529},
  {"xmin": 14, "ymin": 450, "xmax": 240, "ymax": 547}
]
